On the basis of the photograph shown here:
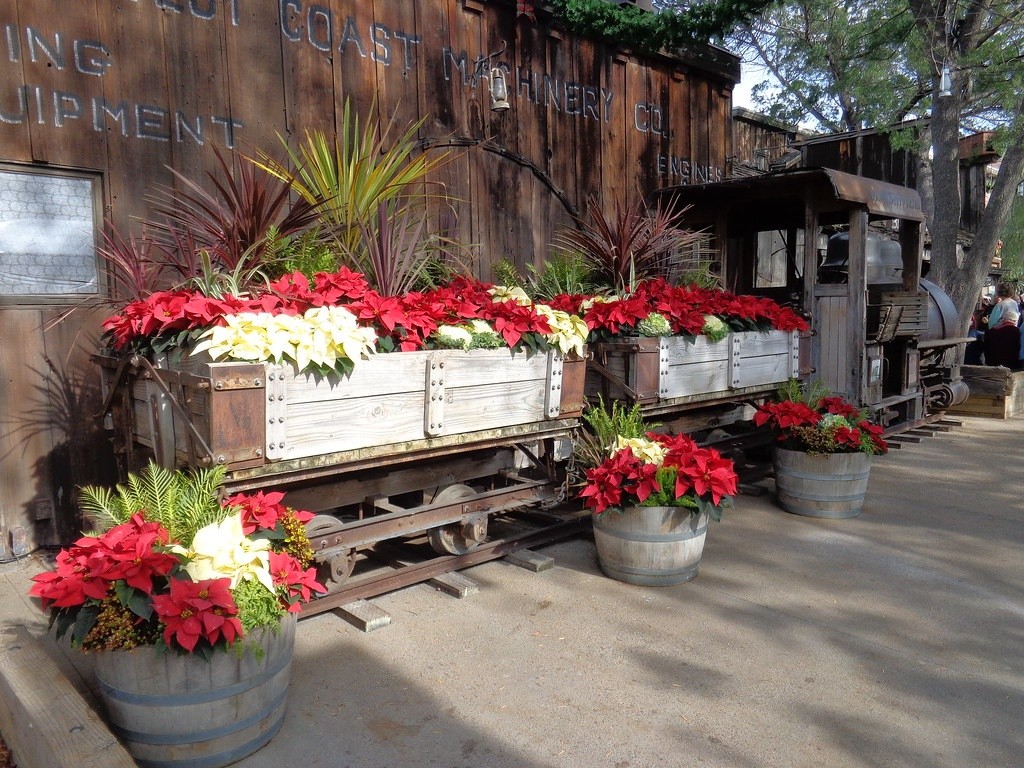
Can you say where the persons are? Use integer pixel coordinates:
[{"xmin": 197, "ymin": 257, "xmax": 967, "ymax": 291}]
[{"xmin": 966, "ymin": 282, "xmax": 1024, "ymax": 371}]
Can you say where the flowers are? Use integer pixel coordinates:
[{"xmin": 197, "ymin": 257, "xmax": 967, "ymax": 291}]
[
  {"xmin": 756, "ymin": 380, "xmax": 890, "ymax": 459},
  {"xmin": 550, "ymin": 182, "xmax": 810, "ymax": 344},
  {"xmin": 94, "ymin": 96, "xmax": 589, "ymax": 369},
  {"xmin": 28, "ymin": 457, "xmax": 330, "ymax": 664},
  {"xmin": 579, "ymin": 391, "xmax": 743, "ymax": 525}
]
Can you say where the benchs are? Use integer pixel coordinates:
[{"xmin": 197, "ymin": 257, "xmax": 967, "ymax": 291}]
[{"xmin": 0, "ymin": 625, "xmax": 137, "ymax": 768}]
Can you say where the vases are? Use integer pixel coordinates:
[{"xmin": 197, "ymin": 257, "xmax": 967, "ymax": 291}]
[
  {"xmin": 592, "ymin": 502, "xmax": 703, "ymax": 584},
  {"xmin": 94, "ymin": 610, "xmax": 297, "ymax": 768},
  {"xmin": 586, "ymin": 327, "xmax": 799, "ymax": 399},
  {"xmin": 773, "ymin": 447, "xmax": 869, "ymax": 520},
  {"xmin": 98, "ymin": 340, "xmax": 589, "ymax": 470}
]
